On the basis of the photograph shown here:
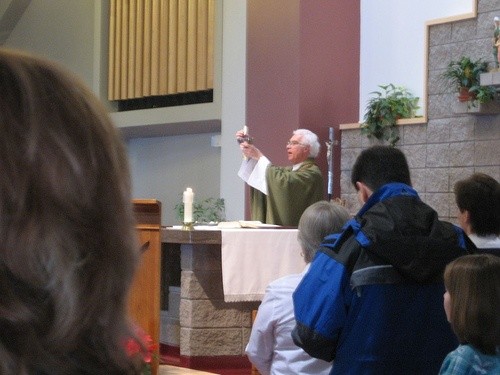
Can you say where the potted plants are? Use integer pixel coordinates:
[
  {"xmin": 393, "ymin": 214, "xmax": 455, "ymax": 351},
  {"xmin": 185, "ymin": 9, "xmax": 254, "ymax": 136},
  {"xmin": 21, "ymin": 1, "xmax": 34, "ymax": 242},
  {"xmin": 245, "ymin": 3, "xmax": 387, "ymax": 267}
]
[
  {"xmin": 438, "ymin": 56, "xmax": 494, "ymax": 106},
  {"xmin": 360, "ymin": 83, "xmax": 421, "ymax": 149}
]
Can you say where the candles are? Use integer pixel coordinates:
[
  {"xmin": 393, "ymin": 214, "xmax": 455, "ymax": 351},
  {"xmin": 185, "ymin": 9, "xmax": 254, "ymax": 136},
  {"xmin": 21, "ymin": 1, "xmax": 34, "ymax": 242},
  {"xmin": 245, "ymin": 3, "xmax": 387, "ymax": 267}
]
[
  {"xmin": 328, "ymin": 127, "xmax": 333, "ymax": 194},
  {"xmin": 244, "ymin": 126, "xmax": 247, "ymax": 135},
  {"xmin": 183, "ymin": 187, "xmax": 194, "ymax": 222}
]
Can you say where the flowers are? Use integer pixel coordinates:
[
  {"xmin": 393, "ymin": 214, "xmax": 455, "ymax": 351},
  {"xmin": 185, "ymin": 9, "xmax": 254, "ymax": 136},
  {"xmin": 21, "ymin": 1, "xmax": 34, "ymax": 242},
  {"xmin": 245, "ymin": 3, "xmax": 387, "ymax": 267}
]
[{"xmin": 126, "ymin": 329, "xmax": 159, "ymax": 375}]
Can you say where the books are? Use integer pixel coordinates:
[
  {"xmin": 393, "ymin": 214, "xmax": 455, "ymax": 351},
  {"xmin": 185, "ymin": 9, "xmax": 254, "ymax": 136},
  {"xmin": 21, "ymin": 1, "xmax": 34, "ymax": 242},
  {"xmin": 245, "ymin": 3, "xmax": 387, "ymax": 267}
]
[{"xmin": 218, "ymin": 220, "xmax": 283, "ymax": 229}]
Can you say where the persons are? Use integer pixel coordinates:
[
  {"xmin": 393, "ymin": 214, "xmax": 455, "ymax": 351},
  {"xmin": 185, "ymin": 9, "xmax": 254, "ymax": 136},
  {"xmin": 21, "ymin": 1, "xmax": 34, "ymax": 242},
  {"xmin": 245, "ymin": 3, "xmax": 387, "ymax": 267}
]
[
  {"xmin": 451, "ymin": 171, "xmax": 500, "ymax": 256},
  {"xmin": 245, "ymin": 201, "xmax": 353, "ymax": 375},
  {"xmin": 438, "ymin": 253, "xmax": 500, "ymax": 375},
  {"xmin": 0, "ymin": 46, "xmax": 162, "ymax": 375},
  {"xmin": 235, "ymin": 124, "xmax": 323, "ymax": 226},
  {"xmin": 291, "ymin": 145, "xmax": 478, "ymax": 375}
]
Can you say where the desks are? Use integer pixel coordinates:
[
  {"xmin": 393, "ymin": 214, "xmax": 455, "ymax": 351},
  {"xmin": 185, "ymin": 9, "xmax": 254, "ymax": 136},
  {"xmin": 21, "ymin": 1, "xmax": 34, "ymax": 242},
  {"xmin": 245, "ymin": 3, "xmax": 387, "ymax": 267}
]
[{"xmin": 126, "ymin": 199, "xmax": 161, "ymax": 375}]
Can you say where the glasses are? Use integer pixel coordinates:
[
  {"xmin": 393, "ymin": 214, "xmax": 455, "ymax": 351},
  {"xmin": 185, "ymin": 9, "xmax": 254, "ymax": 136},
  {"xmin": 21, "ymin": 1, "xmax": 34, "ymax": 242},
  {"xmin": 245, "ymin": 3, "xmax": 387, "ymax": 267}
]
[{"xmin": 288, "ymin": 141, "xmax": 307, "ymax": 147}]
